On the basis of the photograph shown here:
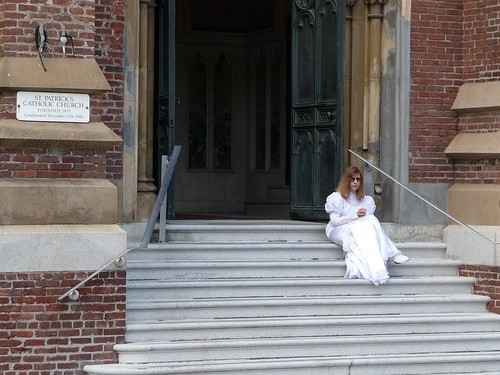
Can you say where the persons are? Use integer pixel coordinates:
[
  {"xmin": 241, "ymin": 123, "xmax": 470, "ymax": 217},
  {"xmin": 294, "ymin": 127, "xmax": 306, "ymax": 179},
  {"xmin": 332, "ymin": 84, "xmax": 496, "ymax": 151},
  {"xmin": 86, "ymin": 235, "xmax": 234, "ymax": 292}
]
[{"xmin": 325, "ymin": 166, "xmax": 409, "ymax": 287}]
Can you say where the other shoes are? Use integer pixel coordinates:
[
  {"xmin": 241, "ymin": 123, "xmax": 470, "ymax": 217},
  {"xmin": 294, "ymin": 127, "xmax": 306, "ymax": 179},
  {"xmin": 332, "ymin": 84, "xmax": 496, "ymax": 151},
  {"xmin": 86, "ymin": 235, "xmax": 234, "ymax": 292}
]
[{"xmin": 390, "ymin": 253, "xmax": 409, "ymax": 264}]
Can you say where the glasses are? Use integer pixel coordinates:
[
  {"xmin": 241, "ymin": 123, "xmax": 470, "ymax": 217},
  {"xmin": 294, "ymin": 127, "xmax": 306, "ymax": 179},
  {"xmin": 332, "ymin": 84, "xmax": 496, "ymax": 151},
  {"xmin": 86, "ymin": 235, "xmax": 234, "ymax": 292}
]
[{"xmin": 349, "ymin": 177, "xmax": 361, "ymax": 182}]
